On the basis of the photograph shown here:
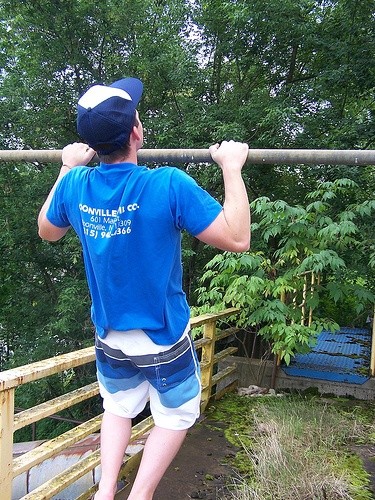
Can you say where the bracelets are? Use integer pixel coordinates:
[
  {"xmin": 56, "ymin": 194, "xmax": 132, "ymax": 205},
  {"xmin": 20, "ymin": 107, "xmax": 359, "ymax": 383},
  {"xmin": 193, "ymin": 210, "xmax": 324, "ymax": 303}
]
[{"xmin": 61, "ymin": 164, "xmax": 72, "ymax": 169}]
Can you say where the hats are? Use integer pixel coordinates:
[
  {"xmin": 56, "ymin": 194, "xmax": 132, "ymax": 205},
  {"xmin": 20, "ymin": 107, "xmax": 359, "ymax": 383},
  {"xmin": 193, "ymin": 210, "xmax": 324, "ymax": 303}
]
[{"xmin": 76, "ymin": 77, "xmax": 144, "ymax": 155}]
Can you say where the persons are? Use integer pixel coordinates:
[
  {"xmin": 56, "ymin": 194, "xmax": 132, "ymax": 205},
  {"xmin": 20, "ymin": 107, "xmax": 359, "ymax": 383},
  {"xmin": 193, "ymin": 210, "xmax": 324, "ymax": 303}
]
[{"xmin": 36, "ymin": 77, "xmax": 252, "ymax": 500}]
